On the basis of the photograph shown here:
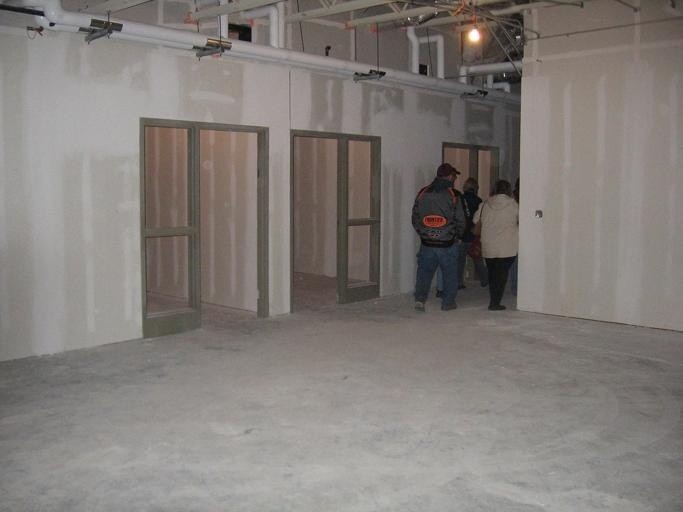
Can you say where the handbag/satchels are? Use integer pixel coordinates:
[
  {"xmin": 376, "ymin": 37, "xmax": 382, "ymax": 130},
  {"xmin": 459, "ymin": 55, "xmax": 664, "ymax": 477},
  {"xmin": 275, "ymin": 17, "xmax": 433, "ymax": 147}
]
[{"xmin": 470, "ymin": 200, "xmax": 487, "ymax": 236}]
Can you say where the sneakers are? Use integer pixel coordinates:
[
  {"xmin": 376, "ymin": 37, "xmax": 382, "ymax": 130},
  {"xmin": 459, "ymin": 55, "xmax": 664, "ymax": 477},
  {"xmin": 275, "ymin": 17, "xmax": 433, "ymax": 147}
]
[
  {"xmin": 436, "ymin": 289, "xmax": 445, "ymax": 298},
  {"xmin": 441, "ymin": 303, "xmax": 456, "ymax": 310},
  {"xmin": 488, "ymin": 304, "xmax": 505, "ymax": 311},
  {"xmin": 415, "ymin": 300, "xmax": 425, "ymax": 310},
  {"xmin": 458, "ymin": 284, "xmax": 465, "ymax": 290}
]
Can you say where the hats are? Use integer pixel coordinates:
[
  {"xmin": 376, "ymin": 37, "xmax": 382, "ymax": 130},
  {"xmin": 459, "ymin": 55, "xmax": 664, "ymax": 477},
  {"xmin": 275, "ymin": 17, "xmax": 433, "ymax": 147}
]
[{"xmin": 437, "ymin": 164, "xmax": 460, "ymax": 176}]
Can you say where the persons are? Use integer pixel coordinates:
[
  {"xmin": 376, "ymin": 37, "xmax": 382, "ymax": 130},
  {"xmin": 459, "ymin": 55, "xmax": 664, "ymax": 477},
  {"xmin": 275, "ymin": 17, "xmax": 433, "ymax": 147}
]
[
  {"xmin": 510, "ymin": 177, "xmax": 519, "ymax": 298},
  {"xmin": 458, "ymin": 177, "xmax": 488, "ymax": 289},
  {"xmin": 435, "ymin": 166, "xmax": 470, "ymax": 299},
  {"xmin": 411, "ymin": 163, "xmax": 467, "ymax": 313},
  {"xmin": 471, "ymin": 179, "xmax": 519, "ymax": 310}
]
